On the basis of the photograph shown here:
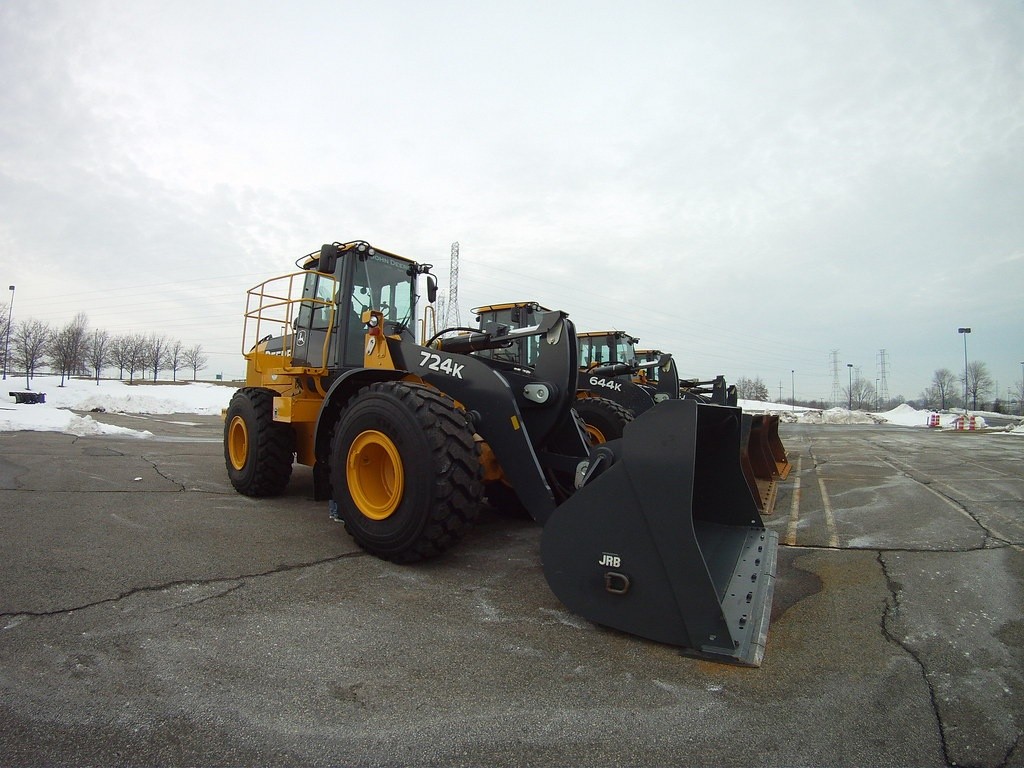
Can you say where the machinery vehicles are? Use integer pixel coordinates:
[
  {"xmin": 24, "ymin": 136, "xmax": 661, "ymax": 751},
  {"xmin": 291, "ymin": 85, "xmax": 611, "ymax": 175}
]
[{"xmin": 223, "ymin": 240, "xmax": 793, "ymax": 668}]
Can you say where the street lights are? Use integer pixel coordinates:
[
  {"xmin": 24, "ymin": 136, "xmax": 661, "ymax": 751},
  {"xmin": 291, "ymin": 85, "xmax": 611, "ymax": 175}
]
[
  {"xmin": 958, "ymin": 328, "xmax": 971, "ymax": 414},
  {"xmin": 847, "ymin": 364, "xmax": 853, "ymax": 410},
  {"xmin": 1021, "ymin": 362, "xmax": 1024, "ymax": 402},
  {"xmin": 791, "ymin": 370, "xmax": 794, "ymax": 411},
  {"xmin": 876, "ymin": 379, "xmax": 879, "ymax": 412},
  {"xmin": 3, "ymin": 286, "xmax": 15, "ymax": 380}
]
